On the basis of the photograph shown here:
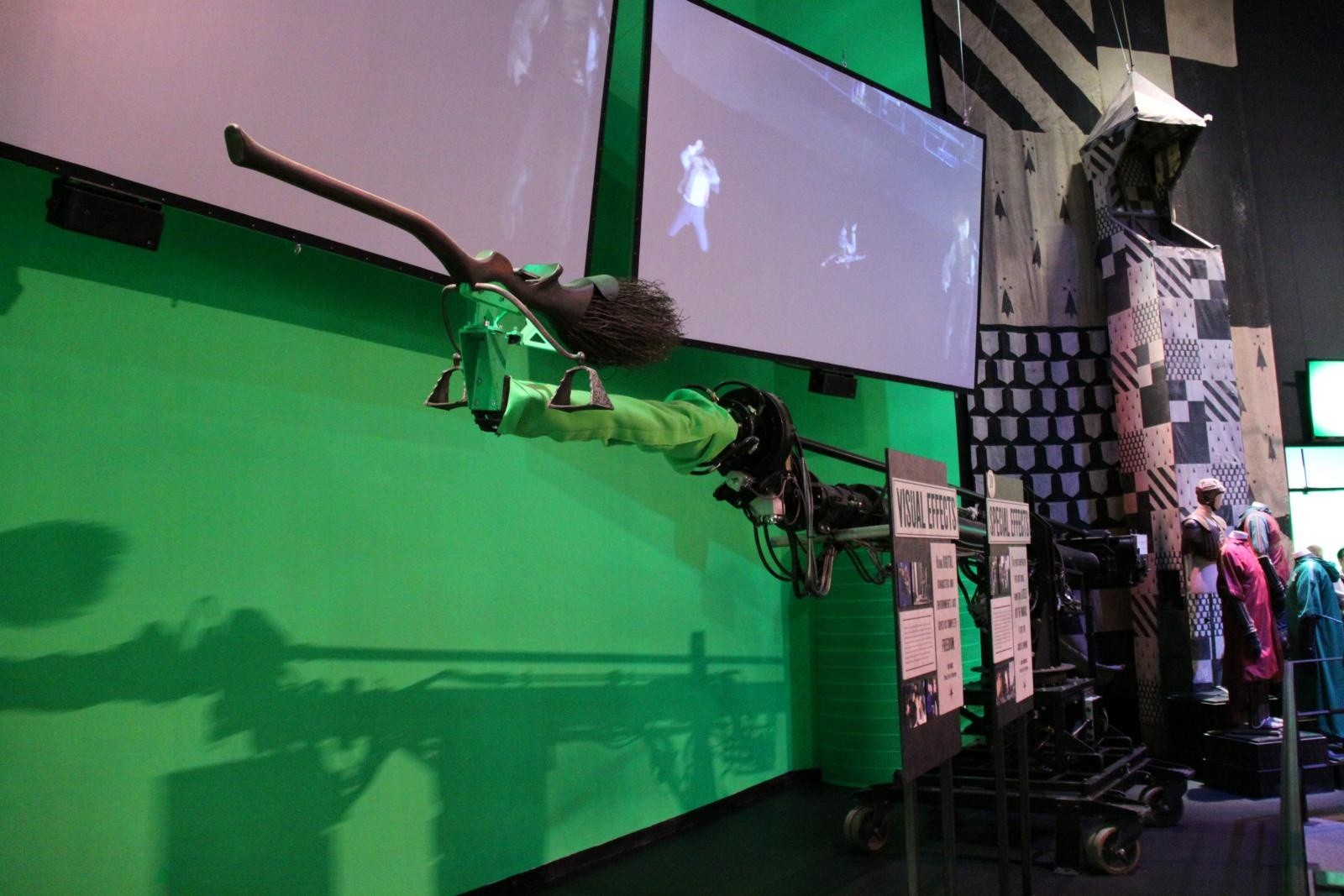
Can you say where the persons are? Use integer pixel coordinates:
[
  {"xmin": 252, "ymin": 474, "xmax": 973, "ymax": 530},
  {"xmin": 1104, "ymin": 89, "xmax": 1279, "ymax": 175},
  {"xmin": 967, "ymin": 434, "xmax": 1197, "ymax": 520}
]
[
  {"xmin": 1179, "ymin": 478, "xmax": 1344, "ymax": 763},
  {"xmin": 938, "ymin": 212, "xmax": 977, "ymax": 368},
  {"xmin": 665, "ymin": 136, "xmax": 719, "ymax": 252},
  {"xmin": 903, "ymin": 677, "xmax": 938, "ymax": 730},
  {"xmin": 504, "ymin": 3, "xmax": 610, "ymax": 266}
]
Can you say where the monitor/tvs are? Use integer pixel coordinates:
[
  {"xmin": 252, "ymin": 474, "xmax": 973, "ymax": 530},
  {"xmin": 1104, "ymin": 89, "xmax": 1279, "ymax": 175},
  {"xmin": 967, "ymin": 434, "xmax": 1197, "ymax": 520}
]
[
  {"xmin": 636, "ymin": 0, "xmax": 990, "ymax": 401},
  {"xmin": 0, "ymin": 0, "xmax": 623, "ymax": 293},
  {"xmin": 1305, "ymin": 357, "xmax": 1344, "ymax": 444}
]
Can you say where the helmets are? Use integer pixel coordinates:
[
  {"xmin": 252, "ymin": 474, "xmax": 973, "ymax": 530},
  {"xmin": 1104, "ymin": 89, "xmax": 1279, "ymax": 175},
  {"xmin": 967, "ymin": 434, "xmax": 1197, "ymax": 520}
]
[{"xmin": 1196, "ymin": 477, "xmax": 1226, "ymax": 512}]
